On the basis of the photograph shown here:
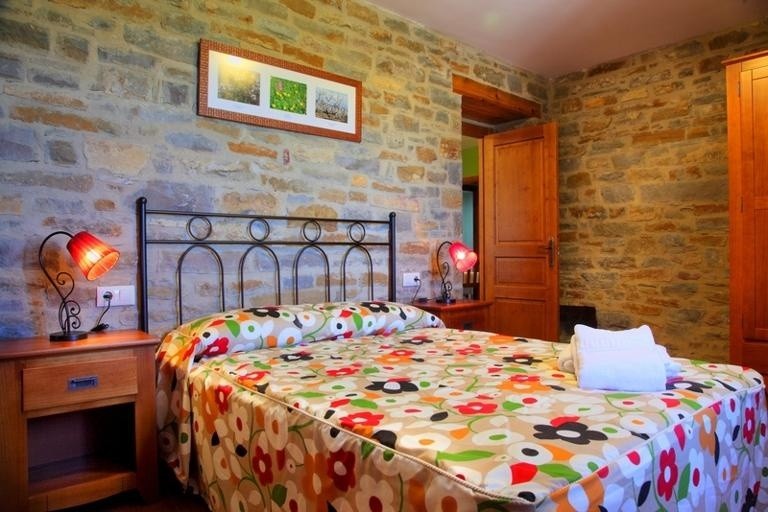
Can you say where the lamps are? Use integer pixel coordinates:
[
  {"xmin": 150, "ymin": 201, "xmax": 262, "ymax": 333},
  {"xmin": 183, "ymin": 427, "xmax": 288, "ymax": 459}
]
[
  {"xmin": 37, "ymin": 230, "xmax": 120, "ymax": 342},
  {"xmin": 436, "ymin": 240, "xmax": 478, "ymax": 303}
]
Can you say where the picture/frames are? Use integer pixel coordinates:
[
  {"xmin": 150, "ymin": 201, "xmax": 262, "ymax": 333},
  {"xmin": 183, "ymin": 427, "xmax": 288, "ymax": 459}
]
[{"xmin": 198, "ymin": 36, "xmax": 363, "ymax": 142}]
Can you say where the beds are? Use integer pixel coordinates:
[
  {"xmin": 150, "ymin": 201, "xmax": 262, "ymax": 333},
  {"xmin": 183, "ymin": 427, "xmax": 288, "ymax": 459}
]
[{"xmin": 135, "ymin": 197, "xmax": 768, "ymax": 512}]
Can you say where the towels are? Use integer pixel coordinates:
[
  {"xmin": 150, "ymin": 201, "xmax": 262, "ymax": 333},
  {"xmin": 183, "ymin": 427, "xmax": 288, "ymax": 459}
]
[
  {"xmin": 558, "ymin": 344, "xmax": 681, "ymax": 377},
  {"xmin": 570, "ymin": 324, "xmax": 667, "ymax": 390}
]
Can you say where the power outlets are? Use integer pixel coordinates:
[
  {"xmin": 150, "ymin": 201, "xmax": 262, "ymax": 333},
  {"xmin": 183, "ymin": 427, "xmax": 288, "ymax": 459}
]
[
  {"xmin": 96, "ymin": 285, "xmax": 136, "ymax": 306},
  {"xmin": 401, "ymin": 272, "xmax": 420, "ymax": 287}
]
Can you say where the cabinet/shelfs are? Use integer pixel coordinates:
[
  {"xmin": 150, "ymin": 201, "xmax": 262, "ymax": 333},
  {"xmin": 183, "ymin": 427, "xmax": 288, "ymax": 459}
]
[
  {"xmin": 0, "ymin": 329, "xmax": 163, "ymax": 512},
  {"xmin": 740, "ymin": 65, "xmax": 768, "ymax": 340},
  {"xmin": 412, "ymin": 296, "xmax": 494, "ymax": 331}
]
